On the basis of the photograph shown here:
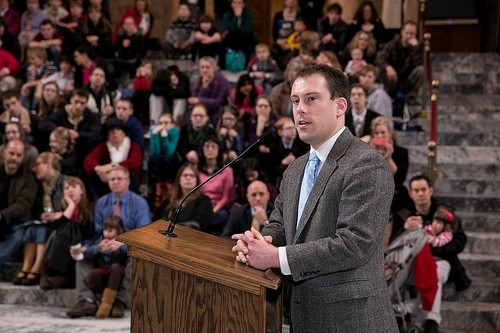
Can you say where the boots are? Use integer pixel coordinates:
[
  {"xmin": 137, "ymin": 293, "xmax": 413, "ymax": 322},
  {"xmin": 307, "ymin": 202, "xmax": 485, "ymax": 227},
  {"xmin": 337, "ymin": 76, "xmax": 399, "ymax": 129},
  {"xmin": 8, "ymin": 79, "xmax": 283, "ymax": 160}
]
[
  {"xmin": 167, "ymin": 183, "xmax": 178, "ymax": 200},
  {"xmin": 156, "ymin": 182, "xmax": 165, "ymax": 208},
  {"xmin": 95, "ymin": 288, "xmax": 117, "ymax": 319}
]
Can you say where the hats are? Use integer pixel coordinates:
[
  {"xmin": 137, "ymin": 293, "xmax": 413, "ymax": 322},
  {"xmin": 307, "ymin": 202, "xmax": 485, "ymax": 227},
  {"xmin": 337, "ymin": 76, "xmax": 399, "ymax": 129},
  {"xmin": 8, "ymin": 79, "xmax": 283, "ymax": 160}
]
[
  {"xmin": 236, "ymin": 73, "xmax": 254, "ymax": 88},
  {"xmin": 99, "ymin": 119, "xmax": 131, "ymax": 136}
]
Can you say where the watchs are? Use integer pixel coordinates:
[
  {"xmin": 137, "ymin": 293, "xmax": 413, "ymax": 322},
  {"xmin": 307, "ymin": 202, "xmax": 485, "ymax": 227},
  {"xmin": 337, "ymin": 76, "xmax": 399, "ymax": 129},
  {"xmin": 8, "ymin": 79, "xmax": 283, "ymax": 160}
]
[{"xmin": 260, "ymin": 221, "xmax": 269, "ymax": 225}]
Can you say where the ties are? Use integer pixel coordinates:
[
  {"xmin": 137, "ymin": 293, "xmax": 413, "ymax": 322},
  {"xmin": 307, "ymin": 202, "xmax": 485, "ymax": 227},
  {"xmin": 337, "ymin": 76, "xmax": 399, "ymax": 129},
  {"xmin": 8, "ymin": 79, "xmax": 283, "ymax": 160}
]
[
  {"xmin": 113, "ymin": 198, "xmax": 120, "ymax": 216},
  {"xmin": 251, "ymin": 217, "xmax": 261, "ymax": 233},
  {"xmin": 296, "ymin": 151, "xmax": 320, "ymax": 231},
  {"xmin": 353, "ymin": 118, "xmax": 362, "ymax": 137}
]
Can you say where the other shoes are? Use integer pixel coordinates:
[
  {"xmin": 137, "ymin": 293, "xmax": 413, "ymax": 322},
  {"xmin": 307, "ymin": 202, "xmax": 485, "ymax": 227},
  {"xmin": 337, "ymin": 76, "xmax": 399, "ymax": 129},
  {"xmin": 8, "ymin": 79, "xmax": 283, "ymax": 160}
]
[
  {"xmin": 407, "ymin": 122, "xmax": 426, "ymax": 133},
  {"xmin": 403, "ymin": 283, "xmax": 418, "ymax": 299},
  {"xmin": 40, "ymin": 276, "xmax": 51, "ymax": 289},
  {"xmin": 19, "ymin": 272, "xmax": 41, "ymax": 286},
  {"xmin": 47, "ymin": 276, "xmax": 70, "ymax": 289},
  {"xmin": 111, "ymin": 303, "xmax": 123, "ymax": 319},
  {"xmin": 392, "ymin": 120, "xmax": 404, "ymax": 133},
  {"xmin": 11, "ymin": 270, "xmax": 28, "ymax": 285},
  {"xmin": 422, "ymin": 319, "xmax": 438, "ymax": 333},
  {"xmin": 66, "ymin": 298, "xmax": 98, "ymax": 318}
]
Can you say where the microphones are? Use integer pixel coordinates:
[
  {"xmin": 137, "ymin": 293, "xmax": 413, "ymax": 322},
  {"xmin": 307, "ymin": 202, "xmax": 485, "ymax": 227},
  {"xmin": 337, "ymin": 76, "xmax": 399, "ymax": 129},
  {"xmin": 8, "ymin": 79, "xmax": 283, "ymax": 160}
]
[{"xmin": 158, "ymin": 127, "xmax": 275, "ymax": 237}]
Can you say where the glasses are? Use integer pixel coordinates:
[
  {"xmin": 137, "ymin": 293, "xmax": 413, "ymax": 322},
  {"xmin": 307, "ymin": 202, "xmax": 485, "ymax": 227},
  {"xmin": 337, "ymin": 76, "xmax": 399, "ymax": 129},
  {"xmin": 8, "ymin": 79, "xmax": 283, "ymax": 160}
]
[
  {"xmin": 159, "ymin": 101, "xmax": 270, "ymax": 178},
  {"xmin": 31, "ymin": 162, "xmax": 45, "ymax": 167}
]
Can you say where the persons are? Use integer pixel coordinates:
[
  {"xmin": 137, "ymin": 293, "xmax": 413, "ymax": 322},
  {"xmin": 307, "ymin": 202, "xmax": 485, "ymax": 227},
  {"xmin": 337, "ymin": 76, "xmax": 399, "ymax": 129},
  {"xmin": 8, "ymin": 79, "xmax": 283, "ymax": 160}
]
[
  {"xmin": 231, "ymin": 63, "xmax": 399, "ymax": 333},
  {"xmin": 0, "ymin": 0, "xmax": 467, "ymax": 333}
]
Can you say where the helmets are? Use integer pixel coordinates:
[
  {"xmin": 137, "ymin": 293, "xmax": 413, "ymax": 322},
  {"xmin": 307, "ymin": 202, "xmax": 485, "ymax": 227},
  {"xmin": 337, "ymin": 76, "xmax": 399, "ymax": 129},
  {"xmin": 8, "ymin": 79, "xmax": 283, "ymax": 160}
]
[{"xmin": 433, "ymin": 209, "xmax": 455, "ymax": 222}]
